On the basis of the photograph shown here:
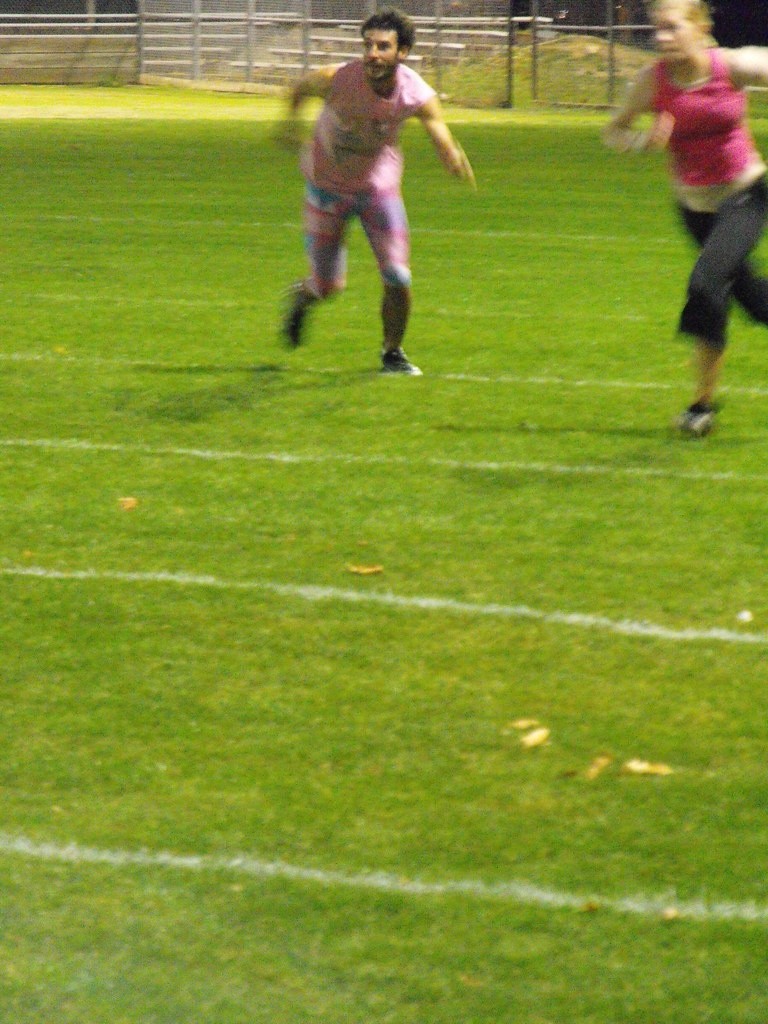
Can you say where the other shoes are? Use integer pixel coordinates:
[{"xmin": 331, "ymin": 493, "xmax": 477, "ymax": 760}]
[
  {"xmin": 282, "ymin": 306, "xmax": 305, "ymax": 346},
  {"xmin": 382, "ymin": 346, "xmax": 423, "ymax": 377},
  {"xmin": 679, "ymin": 400, "xmax": 719, "ymax": 435}
]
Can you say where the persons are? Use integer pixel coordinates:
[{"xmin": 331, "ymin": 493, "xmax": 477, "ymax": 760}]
[
  {"xmin": 276, "ymin": 5, "xmax": 477, "ymax": 377},
  {"xmin": 604, "ymin": 0, "xmax": 768, "ymax": 436}
]
